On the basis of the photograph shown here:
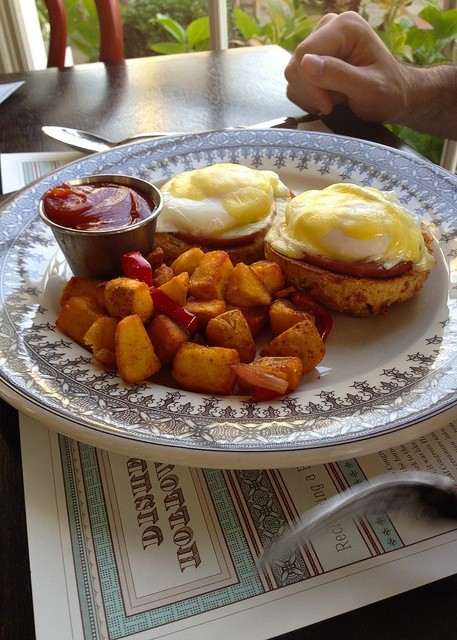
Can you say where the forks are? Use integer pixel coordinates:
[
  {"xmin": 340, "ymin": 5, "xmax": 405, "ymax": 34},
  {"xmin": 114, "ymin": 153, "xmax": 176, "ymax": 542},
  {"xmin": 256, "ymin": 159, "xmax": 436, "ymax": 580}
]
[{"xmin": 253, "ymin": 471, "xmax": 454, "ymax": 569}]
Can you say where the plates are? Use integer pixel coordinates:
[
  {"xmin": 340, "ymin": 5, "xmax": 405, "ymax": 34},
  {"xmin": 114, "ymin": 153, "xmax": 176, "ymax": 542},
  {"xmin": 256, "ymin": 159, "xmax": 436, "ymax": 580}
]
[{"xmin": 0, "ymin": 129, "xmax": 457, "ymax": 469}]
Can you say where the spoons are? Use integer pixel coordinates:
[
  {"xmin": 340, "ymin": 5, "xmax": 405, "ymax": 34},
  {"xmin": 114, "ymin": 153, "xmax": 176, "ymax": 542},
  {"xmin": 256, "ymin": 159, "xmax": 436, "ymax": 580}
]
[{"xmin": 40, "ymin": 111, "xmax": 329, "ymax": 153}]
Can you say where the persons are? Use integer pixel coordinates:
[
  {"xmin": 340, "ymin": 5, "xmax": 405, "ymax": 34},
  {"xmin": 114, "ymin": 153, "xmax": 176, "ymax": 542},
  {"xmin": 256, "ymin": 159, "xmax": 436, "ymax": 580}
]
[{"xmin": 284, "ymin": 10, "xmax": 457, "ymax": 140}]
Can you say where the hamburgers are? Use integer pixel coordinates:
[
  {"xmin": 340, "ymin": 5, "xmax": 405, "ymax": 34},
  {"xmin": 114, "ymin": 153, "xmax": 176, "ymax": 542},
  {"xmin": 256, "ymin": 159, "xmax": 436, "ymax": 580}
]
[
  {"xmin": 155, "ymin": 163, "xmax": 294, "ymax": 265},
  {"xmin": 266, "ymin": 182, "xmax": 437, "ymax": 316}
]
